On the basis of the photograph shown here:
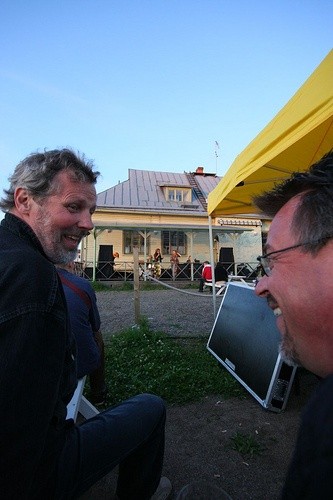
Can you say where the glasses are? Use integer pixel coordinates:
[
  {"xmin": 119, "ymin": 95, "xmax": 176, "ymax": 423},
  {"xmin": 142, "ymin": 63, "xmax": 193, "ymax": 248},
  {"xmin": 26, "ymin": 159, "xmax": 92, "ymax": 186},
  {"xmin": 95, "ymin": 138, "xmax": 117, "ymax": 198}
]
[{"xmin": 256, "ymin": 235, "xmax": 333, "ymax": 277}]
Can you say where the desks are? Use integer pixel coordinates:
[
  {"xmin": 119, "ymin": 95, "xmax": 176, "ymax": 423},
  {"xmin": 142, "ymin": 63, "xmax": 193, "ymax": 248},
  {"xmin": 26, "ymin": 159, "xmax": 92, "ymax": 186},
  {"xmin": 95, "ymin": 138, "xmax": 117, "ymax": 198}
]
[{"xmin": 228, "ymin": 275, "xmax": 246, "ymax": 284}]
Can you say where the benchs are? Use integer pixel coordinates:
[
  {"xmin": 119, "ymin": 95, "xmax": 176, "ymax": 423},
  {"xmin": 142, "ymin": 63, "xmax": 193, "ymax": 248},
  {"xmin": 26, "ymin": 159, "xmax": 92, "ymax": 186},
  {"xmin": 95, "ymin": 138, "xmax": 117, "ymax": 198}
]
[{"xmin": 205, "ymin": 282, "xmax": 227, "ymax": 295}]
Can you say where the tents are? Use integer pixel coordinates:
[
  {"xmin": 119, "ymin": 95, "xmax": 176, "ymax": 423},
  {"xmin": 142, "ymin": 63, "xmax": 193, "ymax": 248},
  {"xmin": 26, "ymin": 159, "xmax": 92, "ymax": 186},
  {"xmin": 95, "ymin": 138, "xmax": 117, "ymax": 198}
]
[{"xmin": 206, "ymin": 40, "xmax": 332, "ymax": 322}]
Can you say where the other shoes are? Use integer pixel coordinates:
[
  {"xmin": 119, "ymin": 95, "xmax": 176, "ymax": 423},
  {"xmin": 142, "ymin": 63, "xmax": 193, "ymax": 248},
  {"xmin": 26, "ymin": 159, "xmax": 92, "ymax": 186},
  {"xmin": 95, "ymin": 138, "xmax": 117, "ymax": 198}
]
[
  {"xmin": 149, "ymin": 477, "xmax": 172, "ymax": 500},
  {"xmin": 88, "ymin": 388, "xmax": 107, "ymax": 405},
  {"xmin": 198, "ymin": 290, "xmax": 203, "ymax": 293}
]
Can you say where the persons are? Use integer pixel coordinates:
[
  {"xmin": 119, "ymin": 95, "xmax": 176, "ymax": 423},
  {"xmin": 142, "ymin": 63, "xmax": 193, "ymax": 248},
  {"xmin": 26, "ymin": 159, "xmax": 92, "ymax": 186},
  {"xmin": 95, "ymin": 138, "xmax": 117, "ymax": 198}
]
[
  {"xmin": 214, "ymin": 261, "xmax": 229, "ymax": 295},
  {"xmin": 186, "ymin": 255, "xmax": 193, "ymax": 278},
  {"xmin": 154, "ymin": 248, "xmax": 164, "ymax": 278},
  {"xmin": 170, "ymin": 249, "xmax": 181, "ymax": 278},
  {"xmin": 198, "ymin": 260, "xmax": 213, "ymax": 294},
  {"xmin": 106, "ymin": 251, "xmax": 120, "ymax": 279},
  {"xmin": 0, "ymin": 147, "xmax": 175, "ymax": 500},
  {"xmin": 254, "ymin": 148, "xmax": 333, "ymax": 500},
  {"xmin": 48, "ymin": 255, "xmax": 111, "ymax": 405}
]
